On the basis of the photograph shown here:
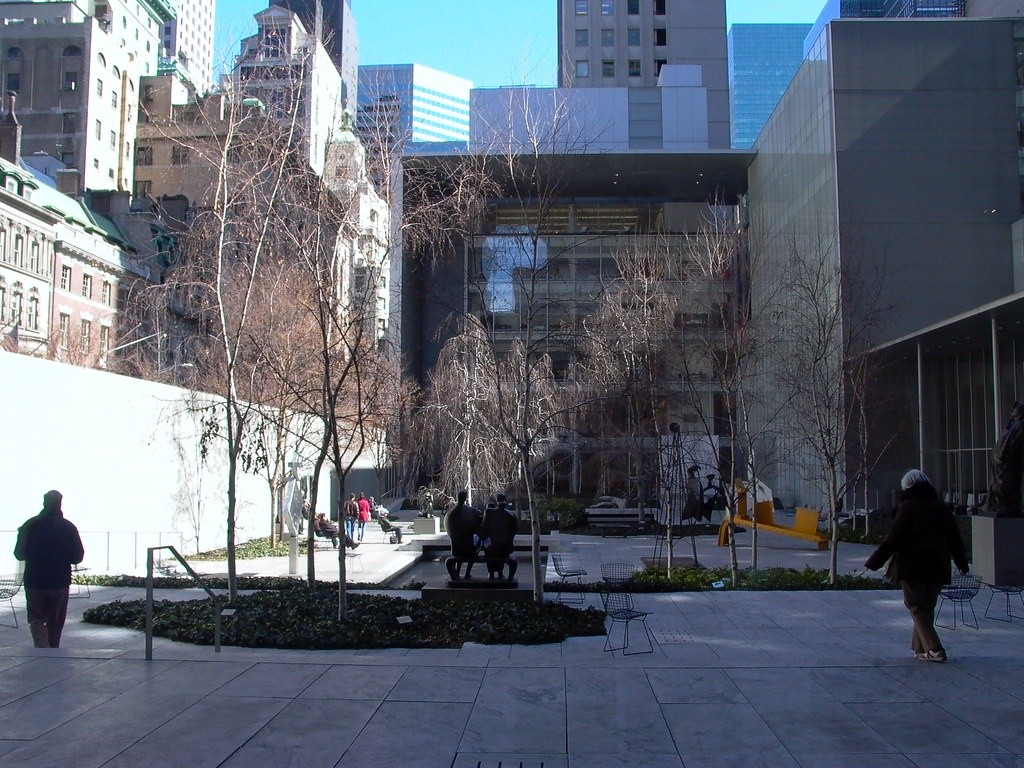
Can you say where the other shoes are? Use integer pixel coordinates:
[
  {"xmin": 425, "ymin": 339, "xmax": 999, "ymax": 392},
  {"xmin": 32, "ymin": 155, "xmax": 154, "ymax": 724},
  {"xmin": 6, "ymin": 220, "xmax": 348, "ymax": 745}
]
[
  {"xmin": 398, "ymin": 541, "xmax": 403, "ymax": 543},
  {"xmin": 351, "ymin": 542, "xmax": 360, "ymax": 550},
  {"xmin": 918, "ymin": 650, "xmax": 945, "ymax": 661}
]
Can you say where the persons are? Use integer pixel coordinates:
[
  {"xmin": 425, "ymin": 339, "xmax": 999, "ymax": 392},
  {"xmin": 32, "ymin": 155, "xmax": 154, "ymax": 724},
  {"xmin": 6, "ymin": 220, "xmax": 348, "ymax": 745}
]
[
  {"xmin": 443, "ymin": 491, "xmax": 483, "ymax": 578},
  {"xmin": 865, "ymin": 467, "xmax": 969, "ymax": 662},
  {"xmin": 482, "ymin": 495, "xmax": 517, "ymax": 580},
  {"xmin": 302, "ymin": 491, "xmax": 404, "ymax": 549},
  {"xmin": 971, "ymin": 400, "xmax": 1024, "ymax": 518},
  {"xmin": 15, "ymin": 490, "xmax": 85, "ymax": 650}
]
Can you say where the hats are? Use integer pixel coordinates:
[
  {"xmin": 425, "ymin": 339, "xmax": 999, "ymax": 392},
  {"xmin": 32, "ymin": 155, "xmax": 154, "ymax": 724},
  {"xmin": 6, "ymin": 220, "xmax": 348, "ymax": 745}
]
[{"xmin": 350, "ymin": 493, "xmax": 356, "ymax": 497}]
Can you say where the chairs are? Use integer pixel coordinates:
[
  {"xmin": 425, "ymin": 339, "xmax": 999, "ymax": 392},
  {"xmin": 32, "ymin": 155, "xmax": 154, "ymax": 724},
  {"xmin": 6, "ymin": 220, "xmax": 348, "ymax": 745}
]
[
  {"xmin": 598, "ymin": 563, "xmax": 654, "ymax": 655},
  {"xmin": 69, "ymin": 563, "xmax": 91, "ymax": 598},
  {"xmin": 314, "ymin": 529, "xmax": 339, "ymax": 550},
  {"xmin": 975, "ymin": 579, "xmax": 1024, "ymax": 623},
  {"xmin": 345, "ymin": 546, "xmax": 364, "ymax": 572},
  {"xmin": 0, "ymin": 573, "xmax": 23, "ymax": 628},
  {"xmin": 381, "ymin": 524, "xmax": 395, "ymax": 544},
  {"xmin": 935, "ymin": 569, "xmax": 982, "ymax": 630},
  {"xmin": 552, "ymin": 553, "xmax": 587, "ymax": 604}
]
